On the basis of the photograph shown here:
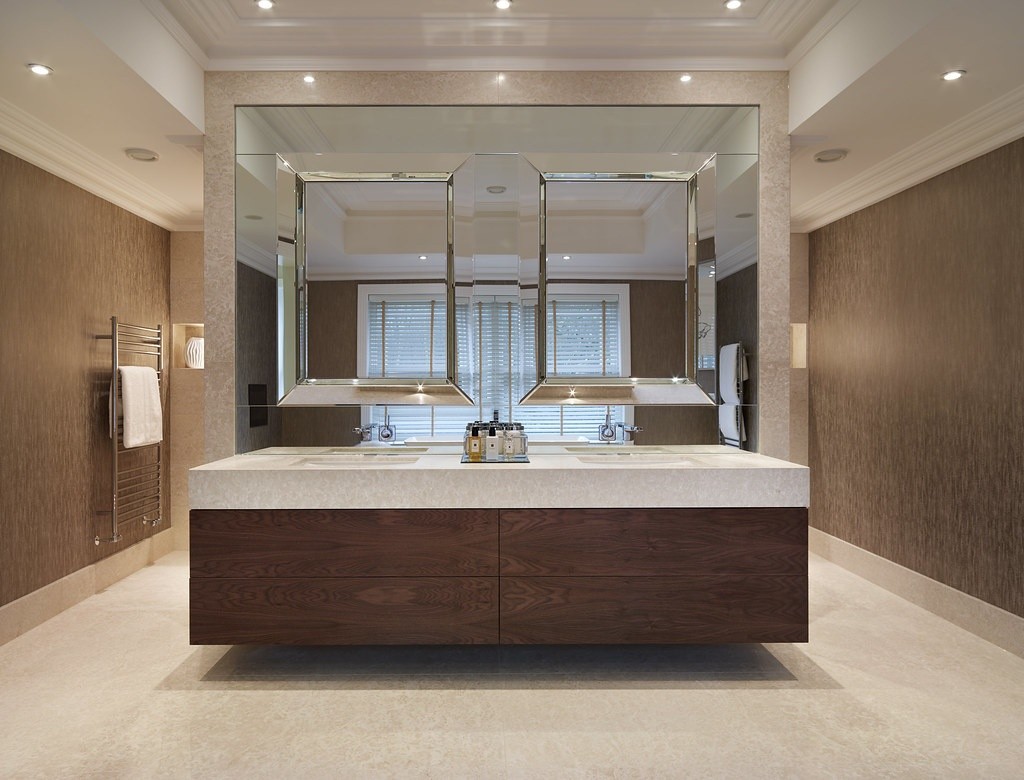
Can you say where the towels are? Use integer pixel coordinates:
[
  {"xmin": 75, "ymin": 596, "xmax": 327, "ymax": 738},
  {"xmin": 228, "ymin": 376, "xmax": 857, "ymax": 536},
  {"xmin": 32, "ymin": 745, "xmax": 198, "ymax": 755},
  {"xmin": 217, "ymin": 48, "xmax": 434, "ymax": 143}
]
[
  {"xmin": 109, "ymin": 366, "xmax": 163, "ymax": 448},
  {"xmin": 719, "ymin": 404, "xmax": 747, "ymax": 449},
  {"xmin": 719, "ymin": 343, "xmax": 748, "ymax": 403}
]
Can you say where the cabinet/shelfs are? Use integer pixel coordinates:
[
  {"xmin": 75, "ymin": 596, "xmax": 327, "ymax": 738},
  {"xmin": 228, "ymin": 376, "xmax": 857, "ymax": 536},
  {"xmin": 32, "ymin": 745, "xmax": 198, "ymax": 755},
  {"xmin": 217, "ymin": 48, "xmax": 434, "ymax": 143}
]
[{"xmin": 190, "ymin": 509, "xmax": 808, "ymax": 644}]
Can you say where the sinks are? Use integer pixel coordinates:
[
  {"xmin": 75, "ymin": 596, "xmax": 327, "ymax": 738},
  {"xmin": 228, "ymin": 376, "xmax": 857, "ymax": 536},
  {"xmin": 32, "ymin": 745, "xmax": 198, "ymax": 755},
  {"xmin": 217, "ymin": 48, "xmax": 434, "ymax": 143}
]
[
  {"xmin": 290, "ymin": 457, "xmax": 419, "ymax": 465},
  {"xmin": 564, "ymin": 446, "xmax": 677, "ymax": 455},
  {"xmin": 573, "ymin": 454, "xmax": 708, "ymax": 466},
  {"xmin": 319, "ymin": 446, "xmax": 429, "ymax": 454}
]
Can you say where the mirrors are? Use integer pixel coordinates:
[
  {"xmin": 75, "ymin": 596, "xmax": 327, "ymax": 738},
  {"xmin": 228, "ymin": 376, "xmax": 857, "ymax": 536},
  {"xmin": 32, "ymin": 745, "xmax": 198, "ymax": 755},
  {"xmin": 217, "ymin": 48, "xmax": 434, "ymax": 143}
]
[
  {"xmin": 537, "ymin": 171, "xmax": 698, "ymax": 384},
  {"xmin": 294, "ymin": 174, "xmax": 455, "ymax": 385}
]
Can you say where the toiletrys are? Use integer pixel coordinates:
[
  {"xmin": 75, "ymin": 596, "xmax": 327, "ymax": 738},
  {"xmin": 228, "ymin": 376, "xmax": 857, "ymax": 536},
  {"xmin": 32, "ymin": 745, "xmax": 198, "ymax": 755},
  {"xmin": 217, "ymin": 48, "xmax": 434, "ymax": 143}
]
[
  {"xmin": 463, "ymin": 420, "xmax": 523, "ymax": 450},
  {"xmin": 466, "ymin": 426, "xmax": 528, "ymax": 461}
]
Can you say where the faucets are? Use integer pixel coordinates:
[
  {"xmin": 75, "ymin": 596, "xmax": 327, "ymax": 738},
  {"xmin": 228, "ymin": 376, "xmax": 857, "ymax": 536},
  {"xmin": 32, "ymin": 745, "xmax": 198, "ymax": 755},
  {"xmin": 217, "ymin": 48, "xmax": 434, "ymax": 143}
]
[
  {"xmin": 352, "ymin": 428, "xmax": 371, "ymax": 440},
  {"xmin": 624, "ymin": 426, "xmax": 643, "ymax": 440}
]
